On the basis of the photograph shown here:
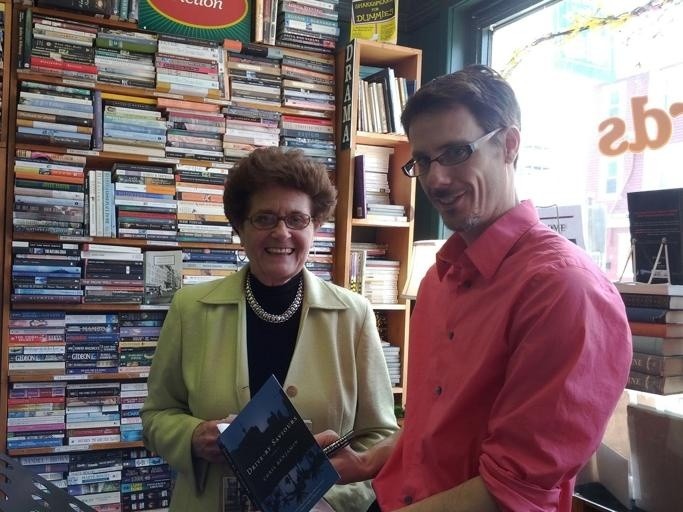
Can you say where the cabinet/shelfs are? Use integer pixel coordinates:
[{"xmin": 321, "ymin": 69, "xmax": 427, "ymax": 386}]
[
  {"xmin": 0, "ymin": 1, "xmax": 338, "ymax": 457},
  {"xmin": 334, "ymin": 37, "xmax": 423, "ymax": 428}
]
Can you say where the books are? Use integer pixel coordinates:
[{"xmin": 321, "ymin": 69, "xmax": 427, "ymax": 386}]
[
  {"xmin": 614, "ymin": 281, "xmax": 683, "ymax": 396},
  {"xmin": 371, "ymin": 310, "xmax": 401, "ymax": 389},
  {"xmin": 350, "ymin": 241, "xmax": 400, "ymax": 305},
  {"xmin": 357, "ymin": 64, "xmax": 416, "ymax": 138},
  {"xmin": 394, "ymin": 406, "xmax": 403, "ymax": 427},
  {"xmin": 22, "ymin": 0, "xmax": 341, "ymax": 53},
  {"xmin": 351, "ymin": 142, "xmax": 408, "ymax": 222},
  {"xmin": 6, "ymin": 17, "xmax": 336, "ymax": 511}
]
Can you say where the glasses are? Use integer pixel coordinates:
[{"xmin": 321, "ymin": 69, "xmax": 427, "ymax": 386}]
[
  {"xmin": 400, "ymin": 127, "xmax": 503, "ymax": 178},
  {"xmin": 244, "ymin": 211, "xmax": 314, "ymax": 230}
]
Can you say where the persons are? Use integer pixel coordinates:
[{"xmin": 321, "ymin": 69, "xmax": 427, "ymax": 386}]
[
  {"xmin": 312, "ymin": 64, "xmax": 634, "ymax": 512},
  {"xmin": 139, "ymin": 145, "xmax": 400, "ymax": 512}
]
